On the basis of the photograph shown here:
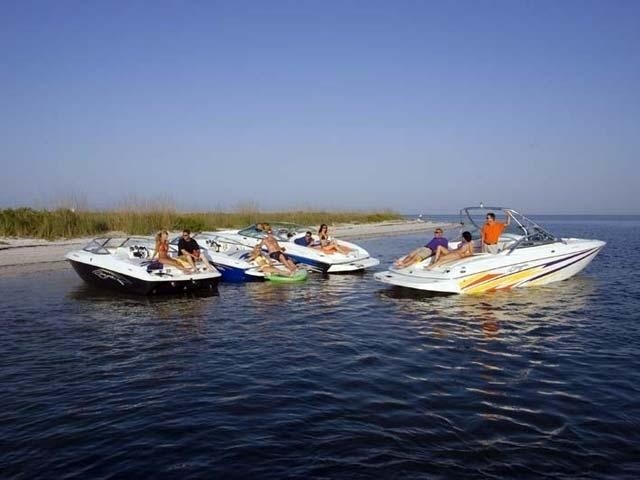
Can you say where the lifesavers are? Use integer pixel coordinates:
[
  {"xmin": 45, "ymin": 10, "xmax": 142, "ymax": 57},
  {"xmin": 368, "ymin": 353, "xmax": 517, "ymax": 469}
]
[{"xmin": 263, "ymin": 268, "xmax": 308, "ymax": 283}]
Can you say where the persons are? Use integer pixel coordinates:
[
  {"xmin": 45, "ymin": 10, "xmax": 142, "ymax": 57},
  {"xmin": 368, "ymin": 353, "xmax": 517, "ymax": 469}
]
[
  {"xmin": 250, "ymin": 244, "xmax": 290, "ymax": 276},
  {"xmin": 479, "ymin": 211, "xmax": 511, "ymax": 254},
  {"xmin": 177, "ymin": 229, "xmax": 215, "ymax": 274},
  {"xmin": 393, "ymin": 228, "xmax": 448, "ymax": 270},
  {"xmin": 318, "ymin": 224, "xmax": 344, "ymax": 253},
  {"xmin": 304, "ymin": 231, "xmax": 321, "ymax": 250},
  {"xmin": 423, "ymin": 231, "xmax": 474, "ymax": 272},
  {"xmin": 150, "ymin": 230, "xmax": 193, "ymax": 275},
  {"xmin": 260, "ymin": 230, "xmax": 297, "ymax": 272}
]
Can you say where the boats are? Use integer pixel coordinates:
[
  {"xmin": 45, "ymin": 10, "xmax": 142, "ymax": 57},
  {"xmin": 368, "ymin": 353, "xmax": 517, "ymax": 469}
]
[{"xmin": 373, "ymin": 206, "xmax": 607, "ymax": 297}]
